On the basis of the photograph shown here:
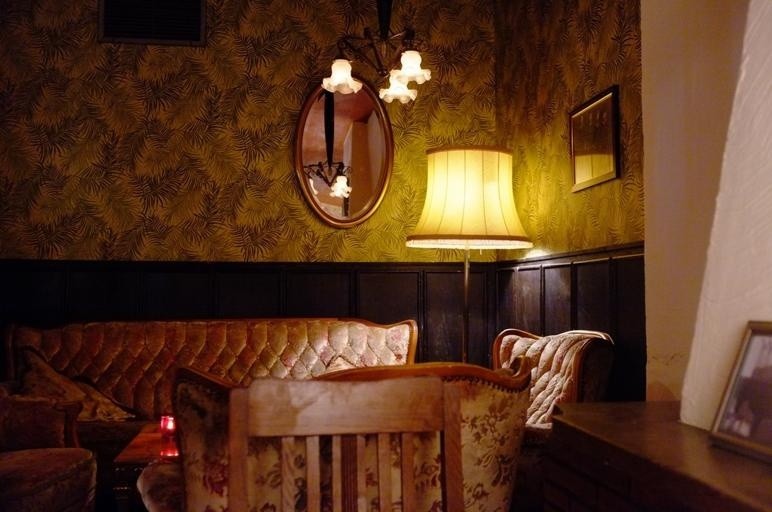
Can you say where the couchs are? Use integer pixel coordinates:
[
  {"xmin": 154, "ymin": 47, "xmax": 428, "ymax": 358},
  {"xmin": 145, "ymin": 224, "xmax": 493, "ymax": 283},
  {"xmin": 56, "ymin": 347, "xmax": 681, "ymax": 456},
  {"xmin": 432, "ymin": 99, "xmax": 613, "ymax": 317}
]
[{"xmin": 2, "ymin": 318, "xmax": 419, "ymax": 512}]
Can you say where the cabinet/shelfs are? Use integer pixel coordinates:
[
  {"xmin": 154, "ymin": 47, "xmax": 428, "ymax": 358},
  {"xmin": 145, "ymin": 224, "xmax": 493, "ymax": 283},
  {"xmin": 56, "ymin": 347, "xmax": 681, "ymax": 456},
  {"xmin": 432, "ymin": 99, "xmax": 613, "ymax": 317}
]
[{"xmin": 539, "ymin": 399, "xmax": 772, "ymax": 512}]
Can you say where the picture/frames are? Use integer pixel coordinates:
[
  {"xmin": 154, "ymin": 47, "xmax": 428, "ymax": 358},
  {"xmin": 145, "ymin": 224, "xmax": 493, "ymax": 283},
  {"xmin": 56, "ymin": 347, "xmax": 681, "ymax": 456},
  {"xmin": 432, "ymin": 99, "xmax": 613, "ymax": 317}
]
[
  {"xmin": 569, "ymin": 85, "xmax": 621, "ymax": 193},
  {"xmin": 708, "ymin": 319, "xmax": 772, "ymax": 466}
]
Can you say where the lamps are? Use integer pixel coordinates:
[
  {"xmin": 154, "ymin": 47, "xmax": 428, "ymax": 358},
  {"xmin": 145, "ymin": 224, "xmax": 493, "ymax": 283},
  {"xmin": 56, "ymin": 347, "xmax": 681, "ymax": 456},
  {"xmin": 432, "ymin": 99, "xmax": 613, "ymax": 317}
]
[
  {"xmin": 405, "ymin": 145, "xmax": 534, "ymax": 363},
  {"xmin": 305, "ymin": 92, "xmax": 354, "ymax": 199},
  {"xmin": 320, "ymin": 1, "xmax": 431, "ymax": 105}
]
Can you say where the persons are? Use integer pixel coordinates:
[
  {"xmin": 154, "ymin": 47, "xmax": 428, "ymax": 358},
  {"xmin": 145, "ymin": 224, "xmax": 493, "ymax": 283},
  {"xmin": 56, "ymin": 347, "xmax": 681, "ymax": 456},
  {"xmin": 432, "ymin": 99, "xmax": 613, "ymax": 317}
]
[{"xmin": 720, "ymin": 395, "xmax": 754, "ymax": 439}]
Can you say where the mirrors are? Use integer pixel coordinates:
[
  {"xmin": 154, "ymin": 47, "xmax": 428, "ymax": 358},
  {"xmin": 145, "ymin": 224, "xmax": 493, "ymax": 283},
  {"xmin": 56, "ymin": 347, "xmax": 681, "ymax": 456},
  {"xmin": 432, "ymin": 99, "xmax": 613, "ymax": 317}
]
[{"xmin": 293, "ymin": 78, "xmax": 394, "ymax": 230}]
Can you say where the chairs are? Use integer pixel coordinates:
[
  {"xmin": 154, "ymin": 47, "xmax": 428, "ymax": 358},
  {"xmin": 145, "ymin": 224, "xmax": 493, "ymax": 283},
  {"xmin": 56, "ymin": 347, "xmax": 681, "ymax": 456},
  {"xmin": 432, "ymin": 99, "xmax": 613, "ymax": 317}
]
[
  {"xmin": 227, "ymin": 376, "xmax": 466, "ymax": 512},
  {"xmin": 137, "ymin": 354, "xmax": 533, "ymax": 512},
  {"xmin": 0, "ymin": 383, "xmax": 98, "ymax": 512},
  {"xmin": 492, "ymin": 327, "xmax": 616, "ymax": 512}
]
